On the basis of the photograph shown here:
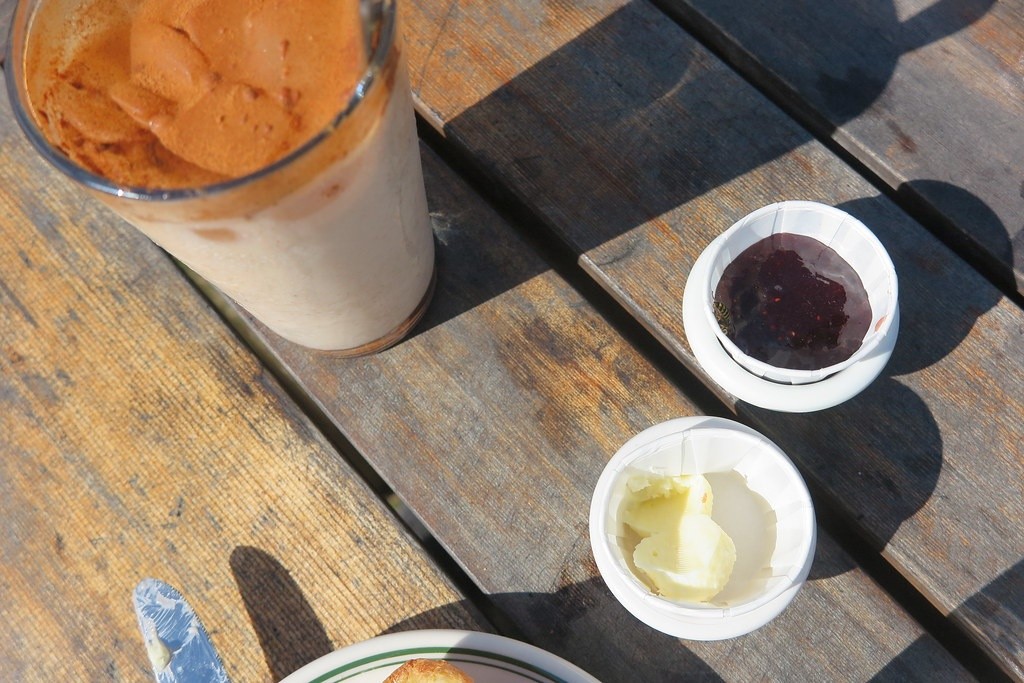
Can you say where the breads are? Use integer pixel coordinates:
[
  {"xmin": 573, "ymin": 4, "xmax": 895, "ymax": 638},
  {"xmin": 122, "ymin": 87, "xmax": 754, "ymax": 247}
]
[{"xmin": 381, "ymin": 658, "xmax": 476, "ymax": 683}]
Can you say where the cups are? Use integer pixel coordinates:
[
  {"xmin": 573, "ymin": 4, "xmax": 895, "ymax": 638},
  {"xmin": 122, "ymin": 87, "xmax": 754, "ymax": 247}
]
[
  {"xmin": 599, "ymin": 429, "xmax": 815, "ymax": 619},
  {"xmin": 707, "ymin": 201, "xmax": 897, "ymax": 383},
  {"xmin": 5, "ymin": 0, "xmax": 437, "ymax": 358}
]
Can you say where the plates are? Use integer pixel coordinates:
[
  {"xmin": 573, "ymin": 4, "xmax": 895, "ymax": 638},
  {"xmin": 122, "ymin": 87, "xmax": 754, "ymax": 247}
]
[
  {"xmin": 275, "ymin": 629, "xmax": 598, "ymax": 683},
  {"xmin": 589, "ymin": 416, "xmax": 817, "ymax": 640},
  {"xmin": 681, "ymin": 199, "xmax": 900, "ymax": 412}
]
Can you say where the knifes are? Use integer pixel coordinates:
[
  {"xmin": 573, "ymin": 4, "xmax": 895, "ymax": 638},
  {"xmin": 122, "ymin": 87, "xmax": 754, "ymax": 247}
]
[{"xmin": 132, "ymin": 579, "xmax": 231, "ymax": 683}]
